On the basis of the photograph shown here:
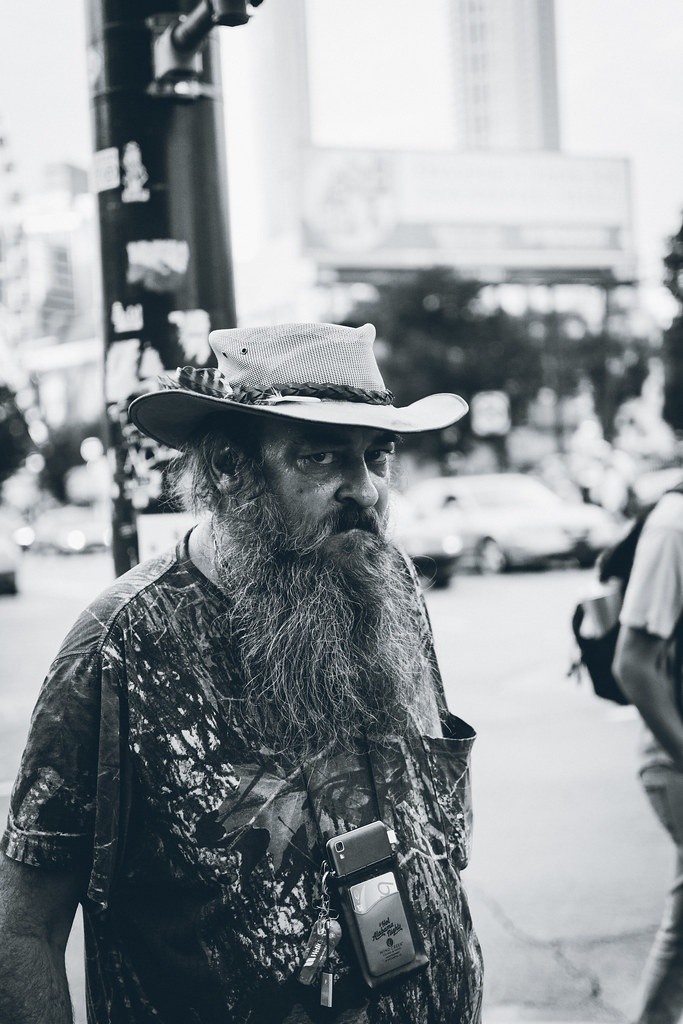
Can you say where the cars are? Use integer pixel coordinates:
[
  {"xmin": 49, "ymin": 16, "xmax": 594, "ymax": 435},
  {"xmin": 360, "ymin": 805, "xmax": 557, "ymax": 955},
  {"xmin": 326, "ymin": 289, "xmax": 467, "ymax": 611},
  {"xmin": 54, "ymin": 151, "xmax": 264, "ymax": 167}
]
[
  {"xmin": 0, "ymin": 506, "xmax": 33, "ymax": 593},
  {"xmin": 388, "ymin": 499, "xmax": 458, "ymax": 588},
  {"xmin": 31, "ymin": 507, "xmax": 112, "ymax": 554},
  {"xmin": 404, "ymin": 474, "xmax": 614, "ymax": 574}
]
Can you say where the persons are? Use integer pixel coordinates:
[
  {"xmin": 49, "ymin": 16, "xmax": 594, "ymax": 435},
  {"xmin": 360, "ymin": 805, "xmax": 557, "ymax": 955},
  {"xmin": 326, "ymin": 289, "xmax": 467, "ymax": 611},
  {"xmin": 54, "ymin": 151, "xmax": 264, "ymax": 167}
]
[
  {"xmin": 0, "ymin": 324, "xmax": 483, "ymax": 1024},
  {"xmin": 611, "ymin": 374, "xmax": 682, "ymax": 1024}
]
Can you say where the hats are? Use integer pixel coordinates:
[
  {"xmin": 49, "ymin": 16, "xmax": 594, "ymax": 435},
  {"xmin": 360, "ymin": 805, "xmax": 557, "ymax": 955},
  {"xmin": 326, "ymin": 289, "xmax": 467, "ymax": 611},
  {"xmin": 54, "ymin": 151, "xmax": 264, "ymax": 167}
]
[{"xmin": 128, "ymin": 306, "xmax": 444, "ymax": 453}]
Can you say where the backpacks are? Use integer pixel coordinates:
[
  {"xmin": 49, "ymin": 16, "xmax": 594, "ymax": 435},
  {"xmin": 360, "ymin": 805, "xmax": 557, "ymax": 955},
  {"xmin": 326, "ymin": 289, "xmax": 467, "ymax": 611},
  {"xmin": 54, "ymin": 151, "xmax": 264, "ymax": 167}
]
[{"xmin": 571, "ymin": 482, "xmax": 683, "ymax": 701}]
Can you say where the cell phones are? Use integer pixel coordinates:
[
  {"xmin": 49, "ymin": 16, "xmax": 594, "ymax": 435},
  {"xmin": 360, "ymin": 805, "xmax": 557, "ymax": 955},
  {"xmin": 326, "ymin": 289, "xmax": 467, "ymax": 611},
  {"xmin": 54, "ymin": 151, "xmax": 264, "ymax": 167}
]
[{"xmin": 326, "ymin": 819, "xmax": 393, "ymax": 877}]
